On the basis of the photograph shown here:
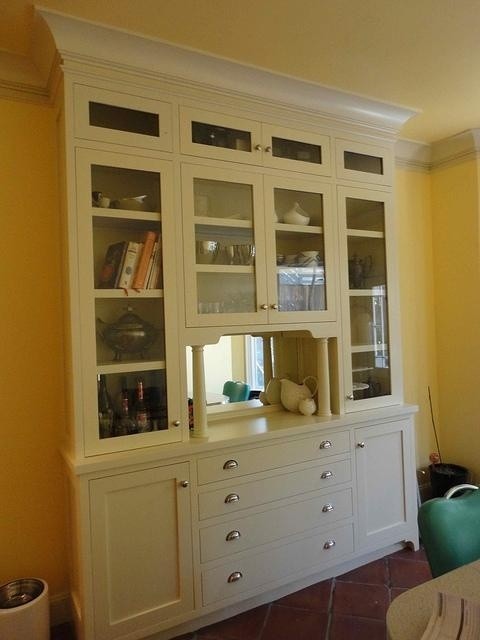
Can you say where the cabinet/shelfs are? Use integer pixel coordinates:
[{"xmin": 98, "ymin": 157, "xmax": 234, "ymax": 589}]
[
  {"xmin": 57, "ymin": 83, "xmax": 174, "ymax": 152},
  {"xmin": 176, "ymin": 104, "xmax": 334, "ymax": 178},
  {"xmin": 61, "ymin": 144, "xmax": 184, "ymax": 459},
  {"xmin": 334, "ymin": 137, "xmax": 394, "ymax": 186},
  {"xmin": 178, "ymin": 158, "xmax": 339, "ymax": 329},
  {"xmin": 186, "ymin": 412, "xmax": 357, "ymax": 629},
  {"xmin": 63, "ymin": 451, "xmax": 197, "ymax": 640},
  {"xmin": 329, "ymin": 184, "xmax": 404, "ymax": 415},
  {"xmin": 343, "ymin": 403, "xmax": 418, "ymax": 569}
]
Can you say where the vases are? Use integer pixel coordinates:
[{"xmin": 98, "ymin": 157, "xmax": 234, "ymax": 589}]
[{"xmin": 430, "ymin": 463, "xmax": 468, "ymax": 497}]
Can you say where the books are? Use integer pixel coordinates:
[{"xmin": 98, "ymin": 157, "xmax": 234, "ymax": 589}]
[{"xmin": 97, "ymin": 231, "xmax": 163, "ymax": 290}]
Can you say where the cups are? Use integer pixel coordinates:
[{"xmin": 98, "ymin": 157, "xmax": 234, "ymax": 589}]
[
  {"xmin": 258, "ymin": 391, "xmax": 265, "ymax": 404},
  {"xmin": 301, "ymin": 399, "xmax": 315, "ymax": 415},
  {"xmin": 198, "ymin": 300, "xmax": 252, "ymax": 312}
]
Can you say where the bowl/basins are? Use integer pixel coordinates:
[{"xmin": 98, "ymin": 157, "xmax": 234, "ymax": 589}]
[{"xmin": 196, "ymin": 240, "xmax": 254, "ymax": 264}]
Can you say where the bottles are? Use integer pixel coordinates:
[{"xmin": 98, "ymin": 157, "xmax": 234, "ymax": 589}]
[
  {"xmin": 98, "ymin": 372, "xmax": 152, "ymax": 439},
  {"xmin": 302, "ymin": 250, "xmax": 320, "ymax": 257},
  {"xmin": 350, "ymin": 251, "xmax": 364, "ymax": 289}
]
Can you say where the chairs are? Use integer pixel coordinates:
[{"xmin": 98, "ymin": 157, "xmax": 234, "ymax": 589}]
[{"xmin": 417, "ymin": 483, "xmax": 480, "ymax": 579}]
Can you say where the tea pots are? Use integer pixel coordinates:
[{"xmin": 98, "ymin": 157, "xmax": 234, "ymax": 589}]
[
  {"xmin": 278, "ymin": 375, "xmax": 318, "ymax": 412},
  {"xmin": 265, "ymin": 376, "xmax": 284, "ymax": 403}
]
